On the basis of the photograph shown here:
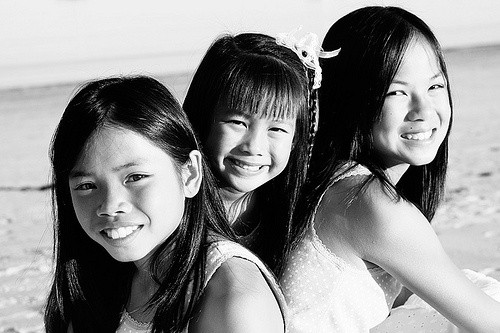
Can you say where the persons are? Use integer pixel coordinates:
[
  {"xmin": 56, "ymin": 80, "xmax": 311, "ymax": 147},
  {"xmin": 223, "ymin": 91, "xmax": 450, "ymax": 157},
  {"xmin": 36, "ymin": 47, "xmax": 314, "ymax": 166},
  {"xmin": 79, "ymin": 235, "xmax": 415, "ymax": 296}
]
[
  {"xmin": 182, "ymin": 24, "xmax": 322, "ymax": 279},
  {"xmin": 43, "ymin": 72, "xmax": 288, "ymax": 333},
  {"xmin": 277, "ymin": 4, "xmax": 499, "ymax": 332}
]
